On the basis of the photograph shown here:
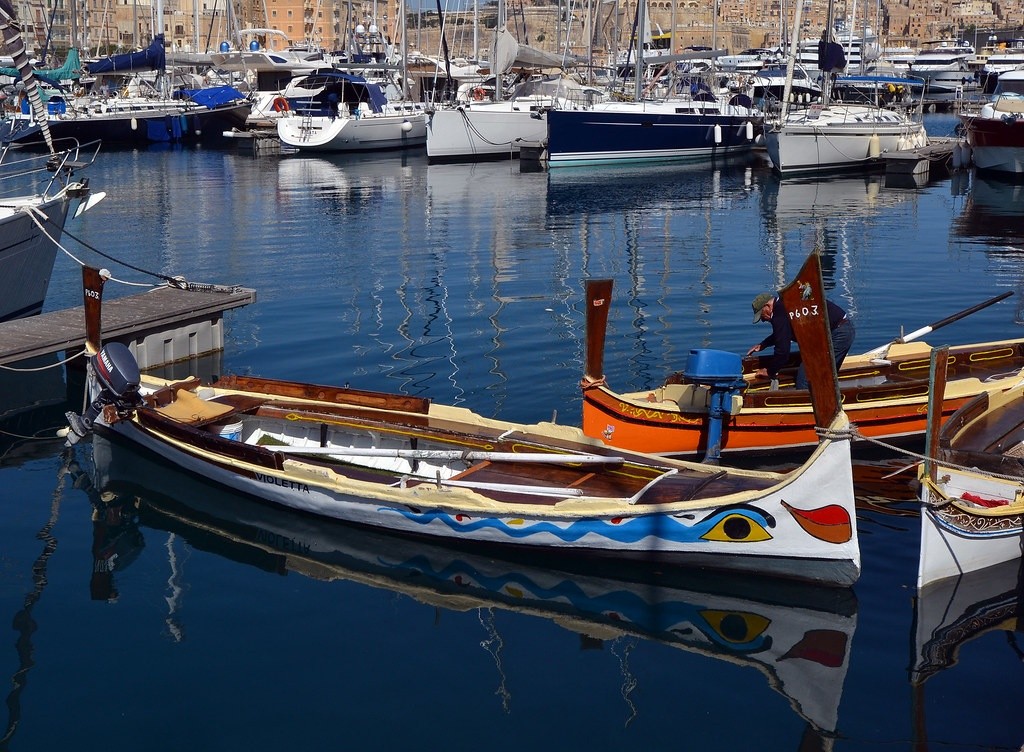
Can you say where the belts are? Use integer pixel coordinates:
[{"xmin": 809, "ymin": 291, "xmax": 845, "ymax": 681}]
[{"xmin": 838, "ymin": 317, "xmax": 849, "ymax": 326}]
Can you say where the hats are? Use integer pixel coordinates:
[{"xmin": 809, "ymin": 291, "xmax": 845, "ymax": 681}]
[{"xmin": 751, "ymin": 292, "xmax": 773, "ymax": 323}]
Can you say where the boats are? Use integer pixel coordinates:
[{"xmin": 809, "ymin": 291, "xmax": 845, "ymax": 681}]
[
  {"xmin": 1, "ymin": 1, "xmax": 1024, "ymax": 323},
  {"xmin": 63, "ymin": 435, "xmax": 861, "ymax": 752},
  {"xmin": 906, "ymin": 557, "xmax": 1023, "ymax": 751},
  {"xmin": 577, "ymin": 276, "xmax": 1024, "ymax": 462},
  {"xmin": 913, "ymin": 342, "xmax": 1024, "ymax": 590},
  {"xmin": 54, "ymin": 238, "xmax": 865, "ymax": 589}
]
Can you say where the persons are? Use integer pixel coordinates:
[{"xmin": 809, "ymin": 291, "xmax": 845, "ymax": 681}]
[
  {"xmin": 746, "ymin": 292, "xmax": 856, "ymax": 391},
  {"xmin": 99, "ymin": 80, "xmax": 128, "ymax": 99},
  {"xmin": 885, "ymin": 82, "xmax": 904, "ymax": 102}
]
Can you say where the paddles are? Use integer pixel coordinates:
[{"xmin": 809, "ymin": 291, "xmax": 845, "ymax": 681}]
[
  {"xmin": 903, "ymin": 287, "xmax": 1014, "ymax": 342},
  {"xmin": 259, "ymin": 451, "xmax": 622, "ymax": 464}
]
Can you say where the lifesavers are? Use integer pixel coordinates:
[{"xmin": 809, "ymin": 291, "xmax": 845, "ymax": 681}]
[
  {"xmin": 274, "ymin": 98, "xmax": 288, "ymax": 112},
  {"xmin": 473, "ymin": 88, "xmax": 485, "ymax": 101}
]
